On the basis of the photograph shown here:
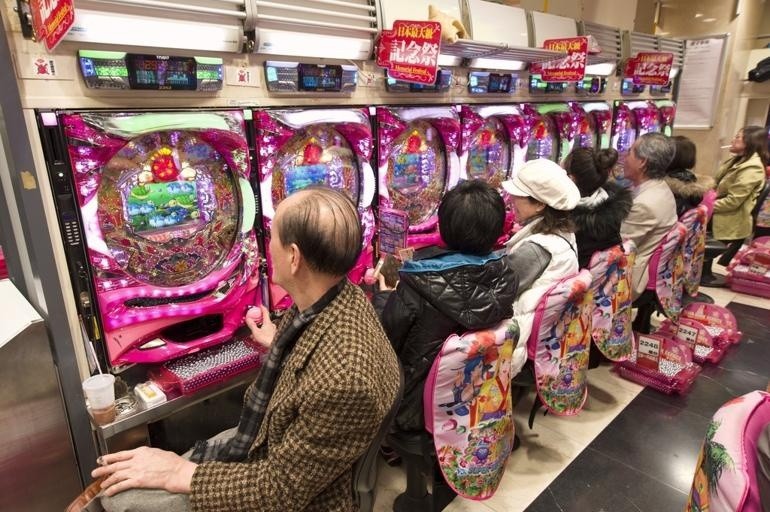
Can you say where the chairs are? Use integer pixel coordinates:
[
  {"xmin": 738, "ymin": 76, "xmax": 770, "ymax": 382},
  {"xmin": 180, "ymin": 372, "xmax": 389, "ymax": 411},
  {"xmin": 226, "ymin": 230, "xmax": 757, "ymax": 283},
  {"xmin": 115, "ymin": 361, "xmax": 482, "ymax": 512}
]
[
  {"xmin": 584, "ymin": 237, "xmax": 639, "ymax": 364},
  {"xmin": 683, "ymin": 185, "xmax": 718, "ymax": 298},
  {"xmin": 347, "ymin": 359, "xmax": 406, "ymax": 511},
  {"xmin": 632, "ymin": 214, "xmax": 700, "ymax": 342},
  {"xmin": 378, "ymin": 316, "xmax": 524, "ymax": 512},
  {"xmin": 683, "ymin": 380, "xmax": 770, "ymax": 512},
  {"xmin": 512, "ymin": 269, "xmax": 601, "ymax": 431}
]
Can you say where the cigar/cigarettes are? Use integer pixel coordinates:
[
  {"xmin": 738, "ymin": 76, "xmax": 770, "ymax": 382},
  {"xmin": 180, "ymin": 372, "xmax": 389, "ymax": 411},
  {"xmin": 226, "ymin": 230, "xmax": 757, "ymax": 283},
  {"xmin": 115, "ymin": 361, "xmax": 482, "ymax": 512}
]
[
  {"xmin": 98, "ymin": 456, "xmax": 107, "ymax": 466},
  {"xmin": 720, "ymin": 145, "xmax": 733, "ymax": 150}
]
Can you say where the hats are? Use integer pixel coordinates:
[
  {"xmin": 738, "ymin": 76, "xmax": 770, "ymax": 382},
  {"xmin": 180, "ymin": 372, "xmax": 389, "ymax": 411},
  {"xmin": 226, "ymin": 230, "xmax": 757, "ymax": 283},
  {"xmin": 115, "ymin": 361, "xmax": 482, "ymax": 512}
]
[{"xmin": 500, "ymin": 158, "xmax": 581, "ymax": 211}]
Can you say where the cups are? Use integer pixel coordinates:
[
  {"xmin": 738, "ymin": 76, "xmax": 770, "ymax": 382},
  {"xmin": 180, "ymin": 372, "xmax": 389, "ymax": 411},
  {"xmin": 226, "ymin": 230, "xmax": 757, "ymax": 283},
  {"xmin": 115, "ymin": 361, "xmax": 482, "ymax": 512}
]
[{"xmin": 82, "ymin": 373, "xmax": 118, "ymax": 425}]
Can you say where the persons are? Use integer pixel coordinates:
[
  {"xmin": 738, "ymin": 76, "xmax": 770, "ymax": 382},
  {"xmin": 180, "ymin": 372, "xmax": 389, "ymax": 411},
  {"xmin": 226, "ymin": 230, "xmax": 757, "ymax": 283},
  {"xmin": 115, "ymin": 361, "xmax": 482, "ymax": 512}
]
[
  {"xmin": 81, "ymin": 187, "xmax": 401, "ymax": 512},
  {"xmin": 709, "ymin": 126, "xmax": 770, "ymax": 242}
]
